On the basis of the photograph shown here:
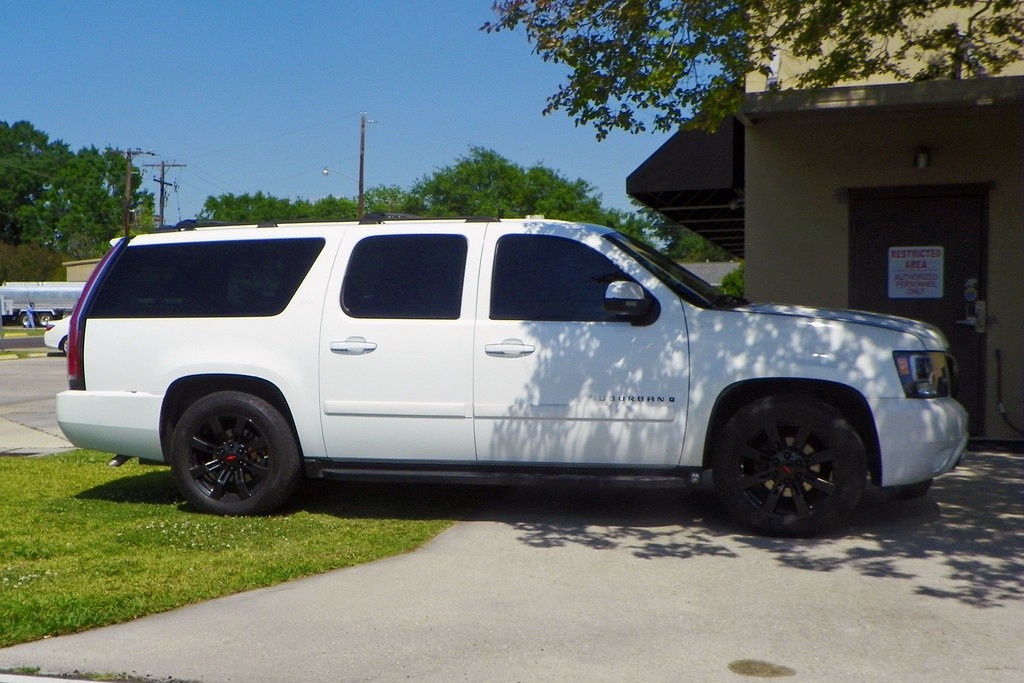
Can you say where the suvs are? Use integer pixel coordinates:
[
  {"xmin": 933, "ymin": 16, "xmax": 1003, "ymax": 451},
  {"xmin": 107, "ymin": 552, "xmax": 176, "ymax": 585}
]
[{"xmin": 54, "ymin": 212, "xmax": 969, "ymax": 538}]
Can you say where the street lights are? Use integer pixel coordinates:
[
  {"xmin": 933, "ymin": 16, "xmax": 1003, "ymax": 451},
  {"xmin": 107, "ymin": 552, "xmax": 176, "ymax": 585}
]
[{"xmin": 323, "ymin": 168, "xmax": 364, "ymax": 221}]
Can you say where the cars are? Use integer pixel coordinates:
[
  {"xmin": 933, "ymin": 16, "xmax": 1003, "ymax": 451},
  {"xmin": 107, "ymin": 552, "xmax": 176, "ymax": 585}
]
[{"xmin": 43, "ymin": 315, "xmax": 71, "ymax": 356}]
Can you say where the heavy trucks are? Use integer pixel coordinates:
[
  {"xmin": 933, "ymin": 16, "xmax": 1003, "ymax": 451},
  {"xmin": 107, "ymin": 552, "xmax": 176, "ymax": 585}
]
[{"xmin": 0, "ymin": 281, "xmax": 87, "ymax": 327}]
